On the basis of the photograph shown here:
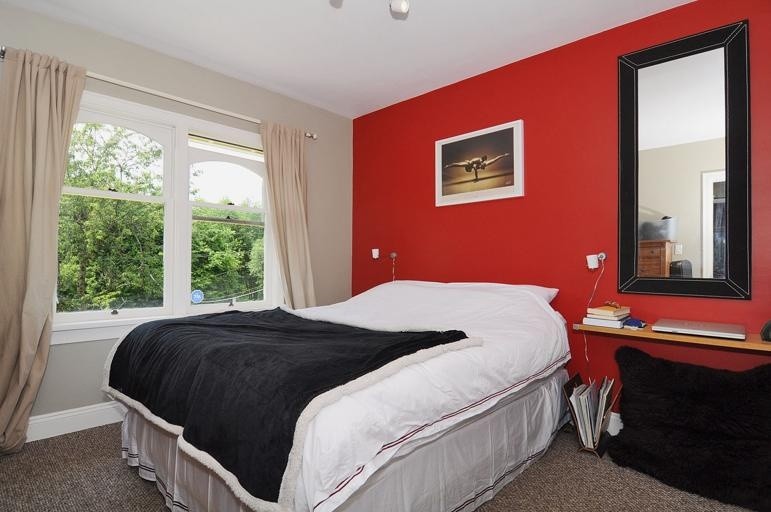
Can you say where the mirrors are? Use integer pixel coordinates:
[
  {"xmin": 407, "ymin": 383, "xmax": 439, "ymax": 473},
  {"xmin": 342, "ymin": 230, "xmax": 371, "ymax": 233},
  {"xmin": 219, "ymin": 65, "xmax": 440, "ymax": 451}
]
[{"xmin": 617, "ymin": 18, "xmax": 752, "ymax": 301}]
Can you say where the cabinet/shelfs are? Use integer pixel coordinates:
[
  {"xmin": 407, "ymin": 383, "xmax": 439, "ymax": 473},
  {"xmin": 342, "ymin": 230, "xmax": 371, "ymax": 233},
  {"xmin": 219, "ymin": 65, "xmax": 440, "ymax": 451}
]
[{"xmin": 637, "ymin": 239, "xmax": 678, "ymax": 278}]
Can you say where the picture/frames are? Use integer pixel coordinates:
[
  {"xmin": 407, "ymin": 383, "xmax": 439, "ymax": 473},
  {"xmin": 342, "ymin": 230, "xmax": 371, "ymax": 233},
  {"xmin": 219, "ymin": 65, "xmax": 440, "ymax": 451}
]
[{"xmin": 434, "ymin": 118, "xmax": 525, "ymax": 207}]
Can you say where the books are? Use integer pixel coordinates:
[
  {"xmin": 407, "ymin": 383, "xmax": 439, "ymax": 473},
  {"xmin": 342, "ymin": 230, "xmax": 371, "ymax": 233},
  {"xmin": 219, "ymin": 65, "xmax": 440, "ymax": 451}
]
[
  {"xmin": 580, "ymin": 302, "xmax": 632, "ymax": 328},
  {"xmin": 569, "ymin": 376, "xmax": 617, "ymax": 448}
]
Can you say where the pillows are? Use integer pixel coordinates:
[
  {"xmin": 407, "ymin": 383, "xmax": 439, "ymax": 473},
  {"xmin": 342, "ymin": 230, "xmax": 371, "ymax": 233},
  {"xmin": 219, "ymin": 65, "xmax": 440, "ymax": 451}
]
[{"xmin": 507, "ymin": 284, "xmax": 560, "ymax": 304}]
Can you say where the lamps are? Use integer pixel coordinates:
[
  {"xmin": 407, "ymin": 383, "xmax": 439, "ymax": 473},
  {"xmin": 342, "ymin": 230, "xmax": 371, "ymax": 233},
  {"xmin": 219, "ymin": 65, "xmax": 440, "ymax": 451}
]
[
  {"xmin": 586, "ymin": 252, "xmax": 606, "ymax": 270},
  {"xmin": 605, "ymin": 345, "xmax": 770, "ymax": 512},
  {"xmin": 371, "ymin": 248, "xmax": 397, "ymax": 259},
  {"xmin": 389, "ymin": 0, "xmax": 409, "ymax": 13}
]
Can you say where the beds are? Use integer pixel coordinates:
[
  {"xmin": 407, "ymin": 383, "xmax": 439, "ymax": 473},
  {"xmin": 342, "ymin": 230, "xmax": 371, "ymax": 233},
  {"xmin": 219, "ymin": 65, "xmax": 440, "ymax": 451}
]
[{"xmin": 123, "ymin": 280, "xmax": 570, "ymax": 512}]
